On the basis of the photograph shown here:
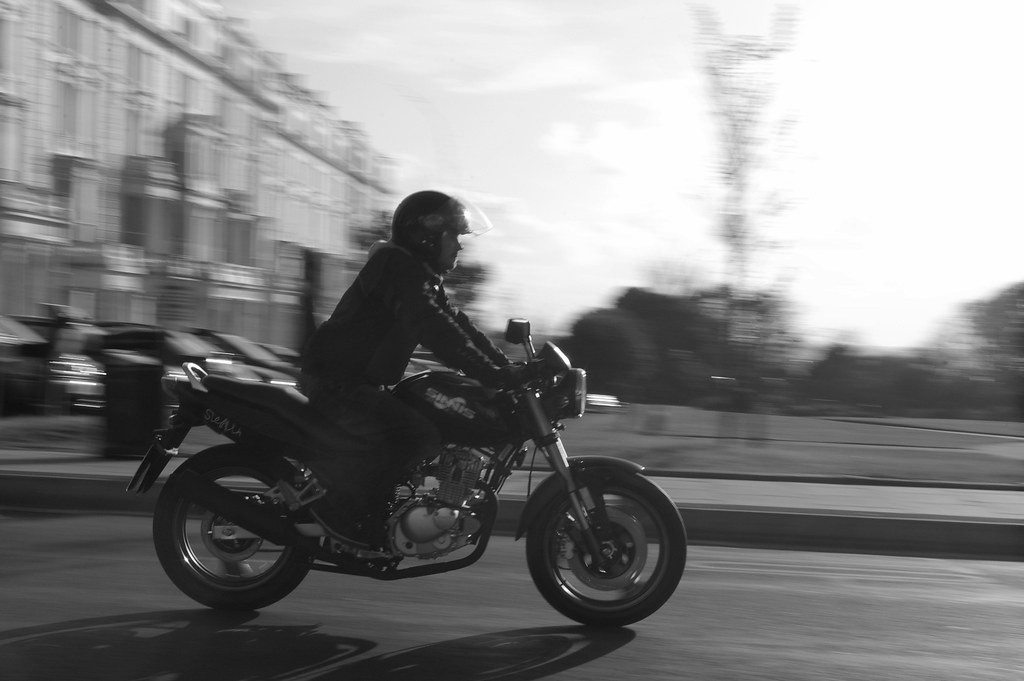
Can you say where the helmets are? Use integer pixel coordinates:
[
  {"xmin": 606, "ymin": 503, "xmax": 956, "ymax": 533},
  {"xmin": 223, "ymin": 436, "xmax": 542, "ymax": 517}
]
[{"xmin": 391, "ymin": 191, "xmax": 472, "ymax": 263}]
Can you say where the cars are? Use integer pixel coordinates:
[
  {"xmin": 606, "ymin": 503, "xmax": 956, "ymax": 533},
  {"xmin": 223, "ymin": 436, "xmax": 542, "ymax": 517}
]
[{"xmin": 1, "ymin": 302, "xmax": 467, "ymax": 445}]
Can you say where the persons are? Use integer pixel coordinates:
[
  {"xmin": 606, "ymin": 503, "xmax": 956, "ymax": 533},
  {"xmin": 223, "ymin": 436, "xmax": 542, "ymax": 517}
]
[{"xmin": 297, "ymin": 189, "xmax": 514, "ymax": 545}]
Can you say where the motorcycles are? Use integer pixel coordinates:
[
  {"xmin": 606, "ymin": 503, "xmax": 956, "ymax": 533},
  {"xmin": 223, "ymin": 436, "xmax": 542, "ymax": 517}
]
[{"xmin": 125, "ymin": 316, "xmax": 689, "ymax": 630}]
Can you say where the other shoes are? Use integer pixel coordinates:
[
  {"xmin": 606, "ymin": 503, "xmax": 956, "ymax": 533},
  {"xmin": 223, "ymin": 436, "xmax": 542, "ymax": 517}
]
[{"xmin": 310, "ymin": 497, "xmax": 373, "ymax": 549}]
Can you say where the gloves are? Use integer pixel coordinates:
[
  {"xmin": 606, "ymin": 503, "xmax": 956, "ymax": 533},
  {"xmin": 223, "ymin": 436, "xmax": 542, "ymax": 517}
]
[{"xmin": 500, "ymin": 366, "xmax": 521, "ymax": 386}]
